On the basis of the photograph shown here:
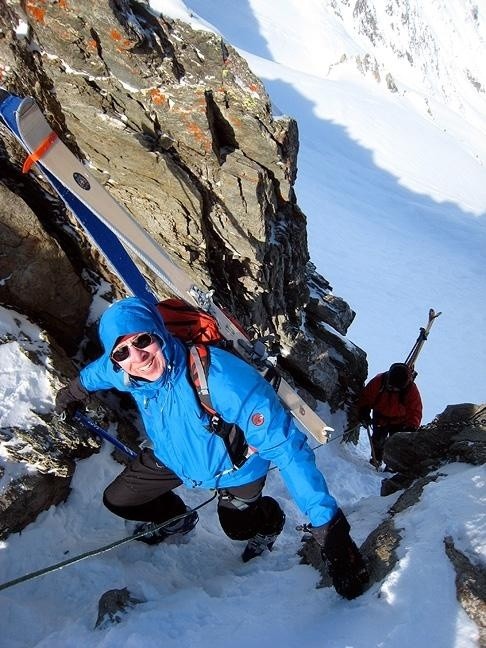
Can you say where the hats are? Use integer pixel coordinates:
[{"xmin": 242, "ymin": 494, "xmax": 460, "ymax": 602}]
[{"xmin": 388, "ymin": 364, "xmax": 411, "ymax": 390}]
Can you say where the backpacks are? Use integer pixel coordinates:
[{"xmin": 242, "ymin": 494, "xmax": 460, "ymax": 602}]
[{"xmin": 156, "ymin": 298, "xmax": 248, "ymax": 471}]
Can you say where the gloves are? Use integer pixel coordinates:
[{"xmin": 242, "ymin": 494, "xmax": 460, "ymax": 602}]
[
  {"xmin": 305, "ymin": 508, "xmax": 370, "ymax": 601},
  {"xmin": 55, "ymin": 377, "xmax": 91, "ymax": 422}
]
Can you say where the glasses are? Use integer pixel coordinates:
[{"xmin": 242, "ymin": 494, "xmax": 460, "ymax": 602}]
[{"xmin": 110, "ymin": 331, "xmax": 151, "ymax": 361}]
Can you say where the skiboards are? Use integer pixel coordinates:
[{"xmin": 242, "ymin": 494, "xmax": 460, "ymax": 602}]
[
  {"xmin": 407, "ymin": 308, "xmax": 441, "ymax": 370},
  {"xmin": 0, "ymin": 86, "xmax": 335, "ymax": 445}
]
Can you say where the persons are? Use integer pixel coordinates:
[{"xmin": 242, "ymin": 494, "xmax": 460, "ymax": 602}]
[
  {"xmin": 356, "ymin": 363, "xmax": 423, "ymax": 475},
  {"xmin": 48, "ymin": 293, "xmax": 373, "ymax": 600}
]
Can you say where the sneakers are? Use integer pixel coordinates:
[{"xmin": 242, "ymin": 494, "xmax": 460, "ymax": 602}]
[
  {"xmin": 242, "ymin": 512, "xmax": 286, "ymax": 564},
  {"xmin": 133, "ymin": 504, "xmax": 198, "ymax": 546}
]
